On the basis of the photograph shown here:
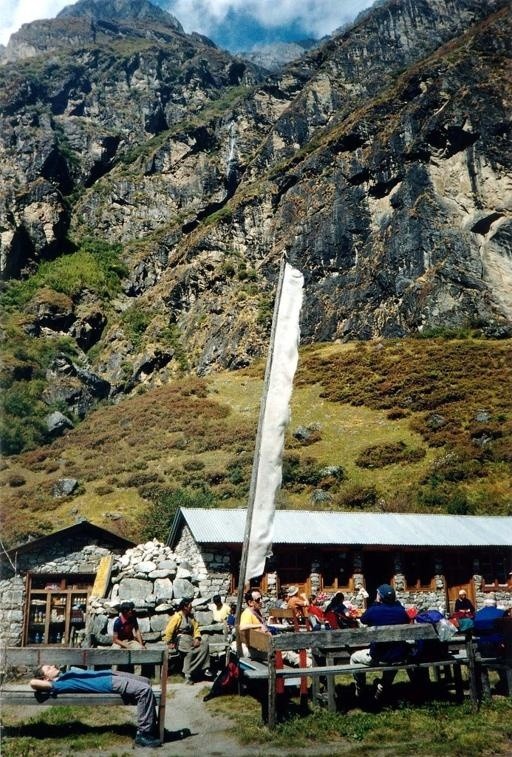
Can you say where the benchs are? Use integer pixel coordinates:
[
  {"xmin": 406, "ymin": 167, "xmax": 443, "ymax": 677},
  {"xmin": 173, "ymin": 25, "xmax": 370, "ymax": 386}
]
[
  {"xmin": 236, "ymin": 608, "xmax": 512, "ymax": 733},
  {"xmin": 0, "ymin": 645, "xmax": 167, "ymax": 745}
]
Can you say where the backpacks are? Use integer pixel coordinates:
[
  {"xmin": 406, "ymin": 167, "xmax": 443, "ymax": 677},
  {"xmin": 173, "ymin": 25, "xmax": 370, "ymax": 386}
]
[{"xmin": 412, "ymin": 611, "xmax": 448, "ymax": 662}]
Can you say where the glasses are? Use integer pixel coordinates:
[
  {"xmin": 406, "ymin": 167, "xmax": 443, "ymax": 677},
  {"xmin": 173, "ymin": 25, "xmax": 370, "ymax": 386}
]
[{"xmin": 251, "ymin": 595, "xmax": 263, "ymax": 603}]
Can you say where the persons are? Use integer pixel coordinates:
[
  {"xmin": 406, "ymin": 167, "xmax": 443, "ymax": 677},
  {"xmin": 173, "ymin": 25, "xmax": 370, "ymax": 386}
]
[
  {"xmin": 110, "ymin": 600, "xmax": 146, "ymax": 675},
  {"xmin": 164, "ymin": 596, "xmax": 214, "ymax": 684},
  {"xmin": 269, "ymin": 580, "xmax": 369, "ymax": 632},
  {"xmin": 222, "ymin": 604, "xmax": 237, "ymax": 642},
  {"xmin": 30, "ymin": 662, "xmax": 162, "ymax": 747},
  {"xmin": 207, "ymin": 594, "xmax": 231, "ymax": 634},
  {"xmin": 349, "ymin": 583, "xmax": 410, "ymax": 701},
  {"xmin": 454, "ymin": 588, "xmax": 475, "ymax": 619},
  {"xmin": 238, "ymin": 587, "xmax": 329, "ymax": 684},
  {"xmin": 470, "ymin": 591, "xmax": 507, "ymax": 682}
]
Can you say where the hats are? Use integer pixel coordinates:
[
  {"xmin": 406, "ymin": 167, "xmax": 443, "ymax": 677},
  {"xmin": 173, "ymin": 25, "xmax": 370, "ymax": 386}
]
[
  {"xmin": 376, "ymin": 583, "xmax": 397, "ymax": 602},
  {"xmin": 458, "ymin": 589, "xmax": 467, "ymax": 596},
  {"xmin": 288, "ymin": 586, "xmax": 299, "ymax": 596}
]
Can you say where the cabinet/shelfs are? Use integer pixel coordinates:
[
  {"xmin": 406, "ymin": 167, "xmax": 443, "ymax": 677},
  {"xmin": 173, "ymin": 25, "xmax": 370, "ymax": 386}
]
[{"xmin": 26, "ymin": 574, "xmax": 93, "ymax": 647}]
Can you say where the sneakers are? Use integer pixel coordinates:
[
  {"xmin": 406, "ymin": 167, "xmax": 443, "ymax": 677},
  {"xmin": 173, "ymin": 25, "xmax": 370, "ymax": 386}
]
[
  {"xmin": 135, "ymin": 731, "xmax": 163, "ymax": 748},
  {"xmin": 182, "ymin": 671, "xmax": 214, "ymax": 686}
]
